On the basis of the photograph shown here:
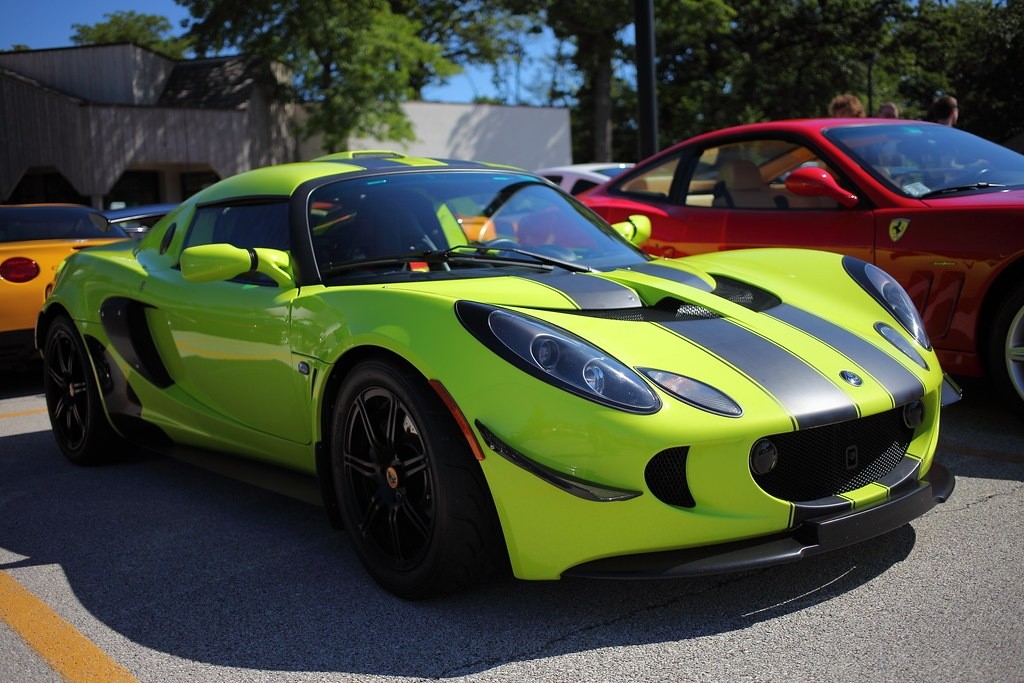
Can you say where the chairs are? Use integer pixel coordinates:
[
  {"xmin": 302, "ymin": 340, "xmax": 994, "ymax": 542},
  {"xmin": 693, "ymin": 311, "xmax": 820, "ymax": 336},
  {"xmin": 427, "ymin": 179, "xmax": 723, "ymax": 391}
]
[{"xmin": 720, "ymin": 158, "xmax": 779, "ymax": 210}]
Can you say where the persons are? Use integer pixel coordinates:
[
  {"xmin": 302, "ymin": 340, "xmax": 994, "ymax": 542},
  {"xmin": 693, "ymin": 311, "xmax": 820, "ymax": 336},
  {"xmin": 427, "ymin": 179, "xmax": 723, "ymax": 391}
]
[
  {"xmin": 921, "ymin": 96, "xmax": 985, "ymax": 167},
  {"xmin": 818, "ymin": 94, "xmax": 868, "ymax": 208},
  {"xmin": 866, "ymin": 102, "xmax": 912, "ymax": 168}
]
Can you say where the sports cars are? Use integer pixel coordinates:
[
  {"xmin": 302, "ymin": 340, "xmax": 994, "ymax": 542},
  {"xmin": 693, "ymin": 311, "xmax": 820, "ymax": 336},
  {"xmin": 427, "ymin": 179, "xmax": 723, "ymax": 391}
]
[{"xmin": 31, "ymin": 151, "xmax": 962, "ymax": 605}]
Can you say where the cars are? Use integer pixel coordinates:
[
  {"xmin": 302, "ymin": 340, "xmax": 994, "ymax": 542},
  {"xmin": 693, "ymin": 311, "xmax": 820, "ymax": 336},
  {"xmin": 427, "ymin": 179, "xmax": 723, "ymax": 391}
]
[
  {"xmin": 514, "ymin": 118, "xmax": 1023, "ymax": 433},
  {"xmin": 1, "ymin": 160, "xmax": 636, "ymax": 356}
]
